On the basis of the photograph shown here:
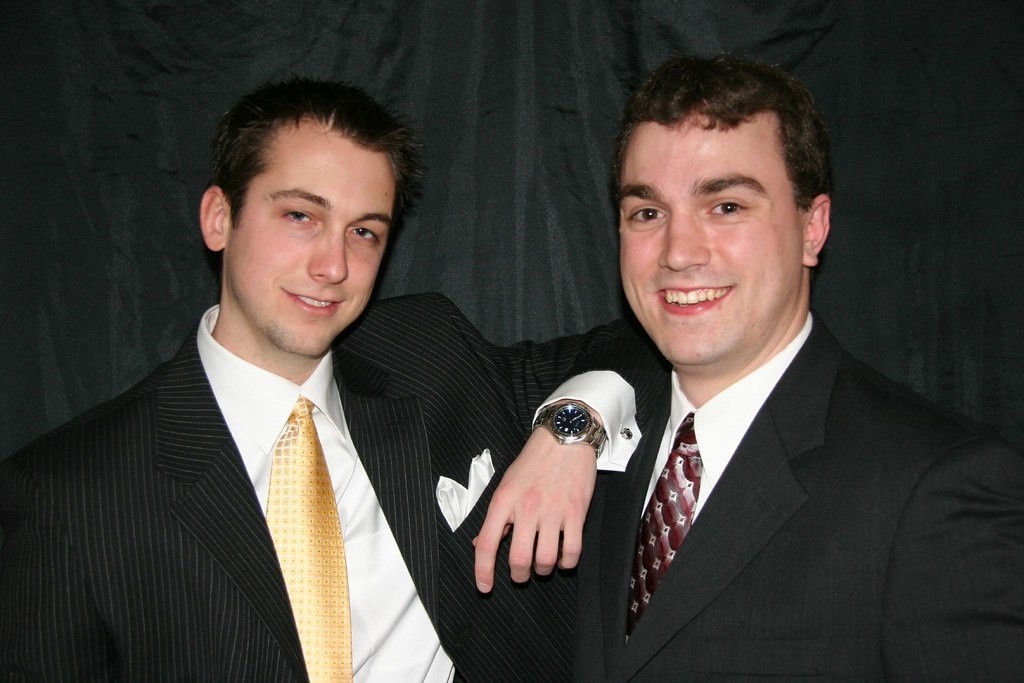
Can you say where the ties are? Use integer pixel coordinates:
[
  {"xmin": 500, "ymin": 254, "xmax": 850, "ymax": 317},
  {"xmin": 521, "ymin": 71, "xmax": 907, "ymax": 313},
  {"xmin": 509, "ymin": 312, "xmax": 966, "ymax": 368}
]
[
  {"xmin": 266, "ymin": 398, "xmax": 353, "ymax": 683},
  {"xmin": 627, "ymin": 412, "xmax": 701, "ymax": 639}
]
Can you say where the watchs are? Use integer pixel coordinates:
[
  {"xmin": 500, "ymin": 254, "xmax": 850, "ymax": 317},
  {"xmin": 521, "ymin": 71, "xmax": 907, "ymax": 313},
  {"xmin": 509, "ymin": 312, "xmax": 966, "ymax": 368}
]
[{"xmin": 533, "ymin": 401, "xmax": 607, "ymax": 459}]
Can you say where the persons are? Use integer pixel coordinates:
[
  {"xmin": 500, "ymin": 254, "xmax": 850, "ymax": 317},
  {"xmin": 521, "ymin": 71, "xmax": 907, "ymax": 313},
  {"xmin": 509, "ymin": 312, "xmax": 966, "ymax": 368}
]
[
  {"xmin": 0, "ymin": 75, "xmax": 675, "ymax": 683},
  {"xmin": 576, "ymin": 54, "xmax": 1024, "ymax": 683}
]
